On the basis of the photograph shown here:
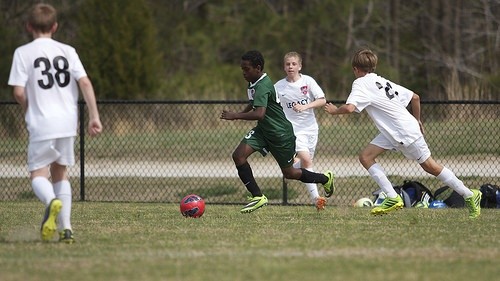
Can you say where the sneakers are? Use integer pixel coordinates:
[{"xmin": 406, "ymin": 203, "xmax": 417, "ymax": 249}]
[
  {"xmin": 59, "ymin": 229, "xmax": 74, "ymax": 244},
  {"xmin": 370, "ymin": 193, "xmax": 404, "ymax": 215},
  {"xmin": 41, "ymin": 198, "xmax": 63, "ymax": 242},
  {"xmin": 464, "ymin": 188, "xmax": 482, "ymax": 218},
  {"xmin": 323, "ymin": 170, "xmax": 334, "ymax": 197},
  {"xmin": 240, "ymin": 194, "xmax": 268, "ymax": 213}
]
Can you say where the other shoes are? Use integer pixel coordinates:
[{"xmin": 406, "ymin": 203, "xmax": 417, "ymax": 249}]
[{"xmin": 317, "ymin": 197, "xmax": 326, "ymax": 212}]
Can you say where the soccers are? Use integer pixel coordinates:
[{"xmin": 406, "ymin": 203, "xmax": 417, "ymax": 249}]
[{"xmin": 180, "ymin": 194, "xmax": 206, "ymax": 218}]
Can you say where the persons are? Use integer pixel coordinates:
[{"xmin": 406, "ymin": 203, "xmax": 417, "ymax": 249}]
[
  {"xmin": 219, "ymin": 50, "xmax": 335, "ymax": 213},
  {"xmin": 8, "ymin": 3, "xmax": 102, "ymax": 242},
  {"xmin": 273, "ymin": 52, "xmax": 327, "ymax": 210},
  {"xmin": 326, "ymin": 49, "xmax": 482, "ymax": 219}
]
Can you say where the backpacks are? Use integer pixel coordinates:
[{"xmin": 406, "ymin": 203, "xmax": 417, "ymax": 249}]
[
  {"xmin": 479, "ymin": 184, "xmax": 500, "ymax": 209},
  {"xmin": 393, "ymin": 180, "xmax": 434, "ymax": 208},
  {"xmin": 434, "ymin": 186, "xmax": 465, "ymax": 208}
]
